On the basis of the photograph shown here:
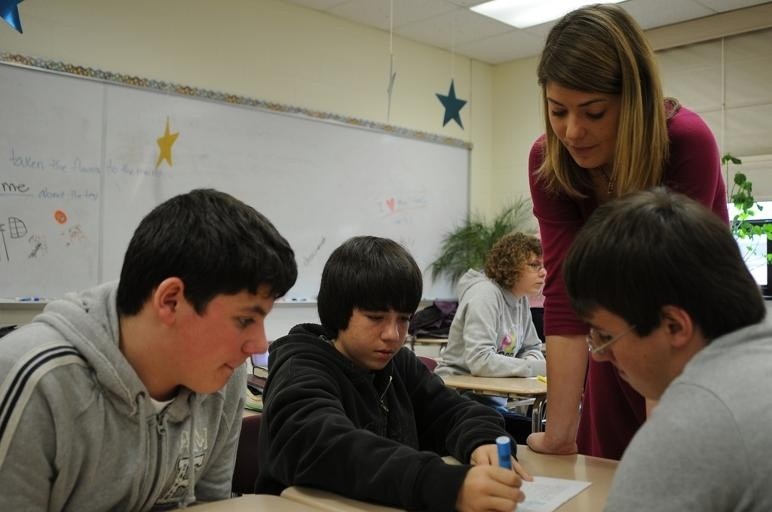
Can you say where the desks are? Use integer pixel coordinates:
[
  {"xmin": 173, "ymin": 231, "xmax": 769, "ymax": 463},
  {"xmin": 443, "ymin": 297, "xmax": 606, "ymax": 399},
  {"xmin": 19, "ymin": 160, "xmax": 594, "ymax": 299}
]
[
  {"xmin": 404, "ymin": 336, "xmax": 447, "ymax": 353},
  {"xmin": 177, "ymin": 444, "xmax": 620, "ymax": 512},
  {"xmin": 444, "ymin": 375, "xmax": 549, "ymax": 432}
]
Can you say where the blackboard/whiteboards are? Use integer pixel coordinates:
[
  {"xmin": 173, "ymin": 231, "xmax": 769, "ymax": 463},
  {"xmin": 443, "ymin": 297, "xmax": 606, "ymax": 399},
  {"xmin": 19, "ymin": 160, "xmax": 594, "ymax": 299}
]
[{"xmin": 0, "ymin": 52, "xmax": 474, "ymax": 313}]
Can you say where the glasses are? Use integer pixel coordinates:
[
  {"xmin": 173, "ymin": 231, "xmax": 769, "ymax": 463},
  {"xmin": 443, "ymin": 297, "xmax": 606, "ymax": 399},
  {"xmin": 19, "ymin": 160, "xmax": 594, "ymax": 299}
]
[
  {"xmin": 584, "ymin": 325, "xmax": 636, "ymax": 360},
  {"xmin": 525, "ymin": 259, "xmax": 545, "ymax": 273}
]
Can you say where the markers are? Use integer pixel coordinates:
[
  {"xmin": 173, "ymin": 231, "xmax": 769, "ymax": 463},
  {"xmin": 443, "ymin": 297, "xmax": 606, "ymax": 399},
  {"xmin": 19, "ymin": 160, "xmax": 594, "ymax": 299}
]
[
  {"xmin": 495, "ymin": 435, "xmax": 512, "ymax": 470},
  {"xmin": 16, "ymin": 297, "xmax": 39, "ymax": 301}
]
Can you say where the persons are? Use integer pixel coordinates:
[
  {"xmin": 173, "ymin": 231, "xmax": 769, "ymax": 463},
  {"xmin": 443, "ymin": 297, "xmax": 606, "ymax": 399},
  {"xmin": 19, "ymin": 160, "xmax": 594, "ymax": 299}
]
[
  {"xmin": 561, "ymin": 187, "xmax": 771, "ymax": 509},
  {"xmin": 257, "ymin": 236, "xmax": 534, "ymax": 509},
  {"xmin": 0, "ymin": 188, "xmax": 298, "ymax": 512},
  {"xmin": 435, "ymin": 233, "xmax": 546, "ymax": 439},
  {"xmin": 525, "ymin": 4, "xmax": 735, "ymax": 460}
]
